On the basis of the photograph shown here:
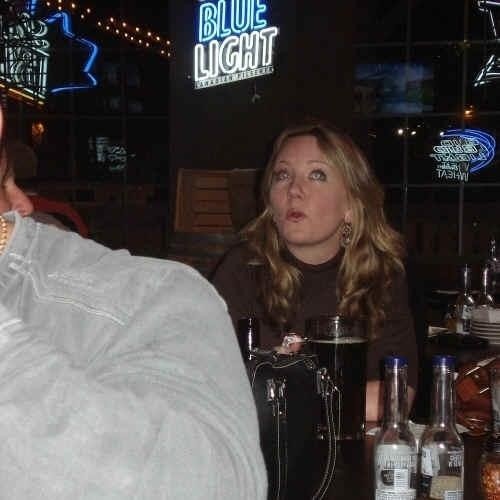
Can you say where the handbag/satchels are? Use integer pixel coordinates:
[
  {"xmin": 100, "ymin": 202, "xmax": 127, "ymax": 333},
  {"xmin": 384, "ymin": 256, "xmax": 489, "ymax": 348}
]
[
  {"xmin": 244, "ymin": 348, "xmax": 342, "ymax": 439},
  {"xmin": 451, "ymin": 353, "xmax": 500, "ymax": 439}
]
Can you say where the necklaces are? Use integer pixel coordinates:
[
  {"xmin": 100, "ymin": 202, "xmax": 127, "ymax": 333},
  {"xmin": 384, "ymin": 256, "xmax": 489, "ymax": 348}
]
[{"xmin": 1, "ymin": 216, "xmax": 8, "ymax": 254}]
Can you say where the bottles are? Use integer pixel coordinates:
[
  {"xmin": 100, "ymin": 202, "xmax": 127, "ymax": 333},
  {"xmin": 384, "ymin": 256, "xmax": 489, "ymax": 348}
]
[
  {"xmin": 369, "ymin": 356, "xmax": 417, "ymax": 500},
  {"xmin": 420, "ymin": 356, "xmax": 467, "ymax": 500},
  {"xmin": 453, "ymin": 263, "xmax": 474, "ymax": 336},
  {"xmin": 476, "ymin": 265, "xmax": 495, "ymax": 306}
]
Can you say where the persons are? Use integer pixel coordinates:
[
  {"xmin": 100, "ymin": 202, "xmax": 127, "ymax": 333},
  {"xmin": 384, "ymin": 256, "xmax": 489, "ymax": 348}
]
[
  {"xmin": 211, "ymin": 118, "xmax": 420, "ymax": 500},
  {"xmin": 1, "ymin": 144, "xmax": 35, "ymax": 217},
  {"xmin": 8, "ymin": 139, "xmax": 90, "ymax": 239},
  {"xmin": 0, "ymin": 106, "xmax": 271, "ymax": 500}
]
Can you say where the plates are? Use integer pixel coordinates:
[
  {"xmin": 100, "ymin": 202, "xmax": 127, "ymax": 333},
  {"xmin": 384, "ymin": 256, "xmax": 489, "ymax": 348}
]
[{"xmin": 468, "ymin": 309, "xmax": 500, "ymax": 341}]
